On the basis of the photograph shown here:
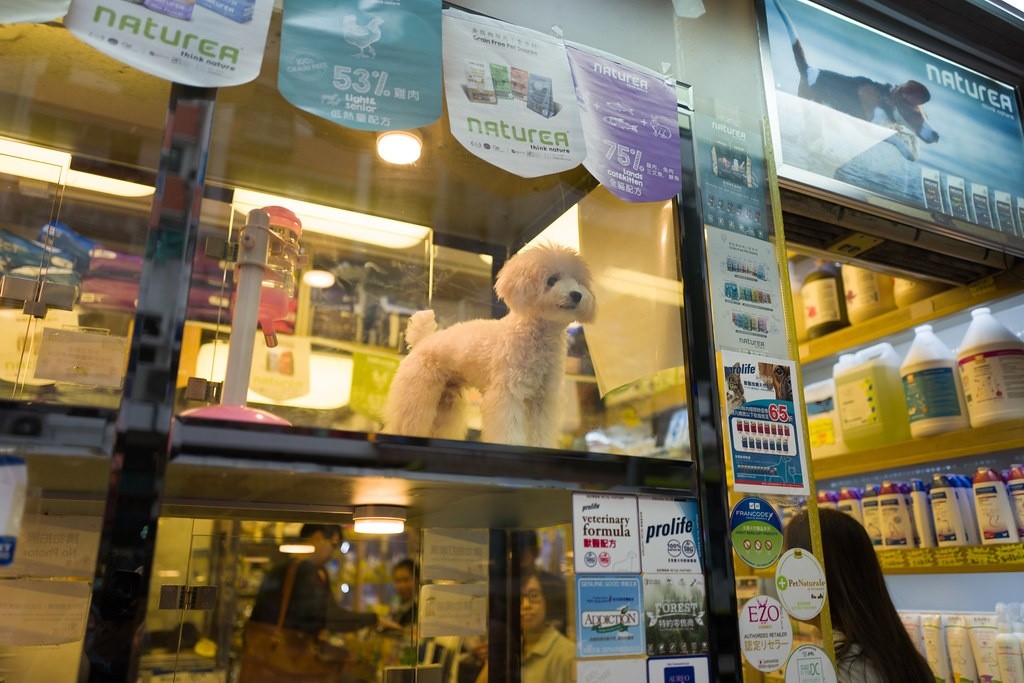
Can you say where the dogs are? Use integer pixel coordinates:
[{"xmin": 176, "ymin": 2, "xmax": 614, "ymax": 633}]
[{"xmin": 383, "ymin": 238, "xmax": 600, "ymax": 451}]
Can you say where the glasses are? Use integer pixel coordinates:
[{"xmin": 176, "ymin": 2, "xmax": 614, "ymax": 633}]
[{"xmin": 519, "ymin": 588, "xmax": 545, "ymax": 606}]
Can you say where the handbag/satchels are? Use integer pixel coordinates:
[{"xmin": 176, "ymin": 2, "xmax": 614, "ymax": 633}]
[{"xmin": 236, "ymin": 621, "xmax": 349, "ymax": 683}]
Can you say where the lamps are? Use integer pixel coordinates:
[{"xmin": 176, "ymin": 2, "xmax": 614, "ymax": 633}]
[{"xmin": 178, "ymin": 185, "xmax": 432, "ymax": 426}]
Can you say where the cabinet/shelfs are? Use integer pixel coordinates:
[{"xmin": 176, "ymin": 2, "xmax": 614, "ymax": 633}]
[{"xmin": 0, "ymin": 0, "xmax": 1024, "ymax": 683}]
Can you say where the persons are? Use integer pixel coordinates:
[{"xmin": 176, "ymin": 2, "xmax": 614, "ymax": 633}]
[
  {"xmin": 478, "ymin": 568, "xmax": 578, "ymax": 683},
  {"xmin": 238, "ymin": 523, "xmax": 406, "ymax": 683},
  {"xmin": 779, "ymin": 507, "xmax": 941, "ymax": 683},
  {"xmin": 442, "ymin": 528, "xmax": 568, "ymax": 683},
  {"xmin": 376, "ymin": 559, "xmax": 418, "ymax": 634}
]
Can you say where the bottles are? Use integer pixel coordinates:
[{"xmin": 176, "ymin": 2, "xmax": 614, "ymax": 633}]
[
  {"xmin": 900, "ymin": 325, "xmax": 971, "ymax": 437},
  {"xmin": 788, "ymin": 261, "xmax": 809, "ymax": 343},
  {"xmin": 800, "ymin": 258, "xmax": 852, "ymax": 339},
  {"xmin": 842, "ymin": 264, "xmax": 897, "ymax": 325},
  {"xmin": 804, "ymin": 379, "xmax": 847, "ymax": 459},
  {"xmin": 893, "ymin": 277, "xmax": 947, "ymax": 308},
  {"xmin": 833, "ymin": 342, "xmax": 912, "ymax": 451},
  {"xmin": 956, "ymin": 307, "xmax": 1024, "ymax": 428},
  {"xmin": 260, "ymin": 206, "xmax": 302, "ymax": 346}
]
